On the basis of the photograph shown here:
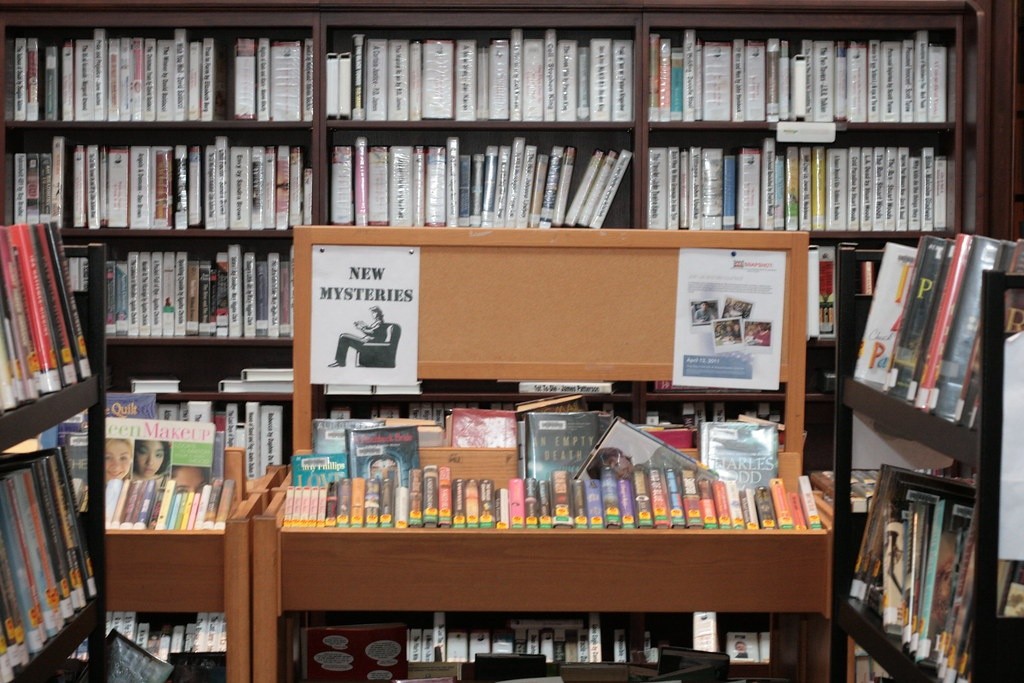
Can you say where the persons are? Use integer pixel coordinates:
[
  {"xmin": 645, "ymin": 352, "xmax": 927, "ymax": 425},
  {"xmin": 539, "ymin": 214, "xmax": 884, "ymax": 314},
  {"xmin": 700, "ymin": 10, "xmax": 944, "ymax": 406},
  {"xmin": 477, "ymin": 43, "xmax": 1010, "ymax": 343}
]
[
  {"xmin": 105, "ymin": 439, "xmax": 132, "ymax": 481},
  {"xmin": 735, "ymin": 642, "xmax": 748, "ymax": 658},
  {"xmin": 587, "ymin": 446, "xmax": 698, "ymax": 483},
  {"xmin": 173, "ymin": 466, "xmax": 209, "ymax": 496},
  {"xmin": 134, "ymin": 440, "xmax": 170, "ymax": 482},
  {"xmin": 694, "ymin": 302, "xmax": 716, "ymax": 322},
  {"xmin": 746, "ymin": 325, "xmax": 757, "ymax": 346},
  {"xmin": 754, "ymin": 323, "xmax": 771, "ymax": 346},
  {"xmin": 715, "ymin": 323, "xmax": 741, "ymax": 341}
]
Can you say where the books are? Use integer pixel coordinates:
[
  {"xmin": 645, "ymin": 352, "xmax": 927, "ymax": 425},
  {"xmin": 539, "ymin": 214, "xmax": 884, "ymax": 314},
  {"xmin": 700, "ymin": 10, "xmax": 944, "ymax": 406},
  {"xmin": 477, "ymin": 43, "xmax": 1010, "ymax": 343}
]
[
  {"xmin": 0, "ymin": 222, "xmax": 98, "ymax": 683},
  {"xmin": 519, "ymin": 379, "xmax": 613, "ymax": 394},
  {"xmin": 287, "ymin": 397, "xmax": 823, "ymax": 531},
  {"xmin": 11, "ymin": 30, "xmax": 949, "ymax": 124},
  {"xmin": 100, "ymin": 367, "xmax": 295, "ymax": 536},
  {"xmin": 105, "ymin": 243, "xmax": 294, "ymax": 338},
  {"xmin": 105, "ymin": 610, "xmax": 227, "ymax": 661},
  {"xmin": 10, "ymin": 137, "xmax": 955, "ymax": 230},
  {"xmin": 810, "ymin": 233, "xmax": 1024, "ymax": 683},
  {"xmin": 301, "ymin": 611, "xmax": 771, "ymax": 683}
]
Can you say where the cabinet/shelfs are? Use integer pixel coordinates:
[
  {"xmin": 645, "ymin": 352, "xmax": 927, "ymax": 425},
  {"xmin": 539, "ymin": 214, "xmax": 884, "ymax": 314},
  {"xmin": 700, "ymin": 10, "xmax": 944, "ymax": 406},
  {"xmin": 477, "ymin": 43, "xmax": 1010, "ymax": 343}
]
[{"xmin": 0, "ymin": 2, "xmax": 1024, "ymax": 683}]
